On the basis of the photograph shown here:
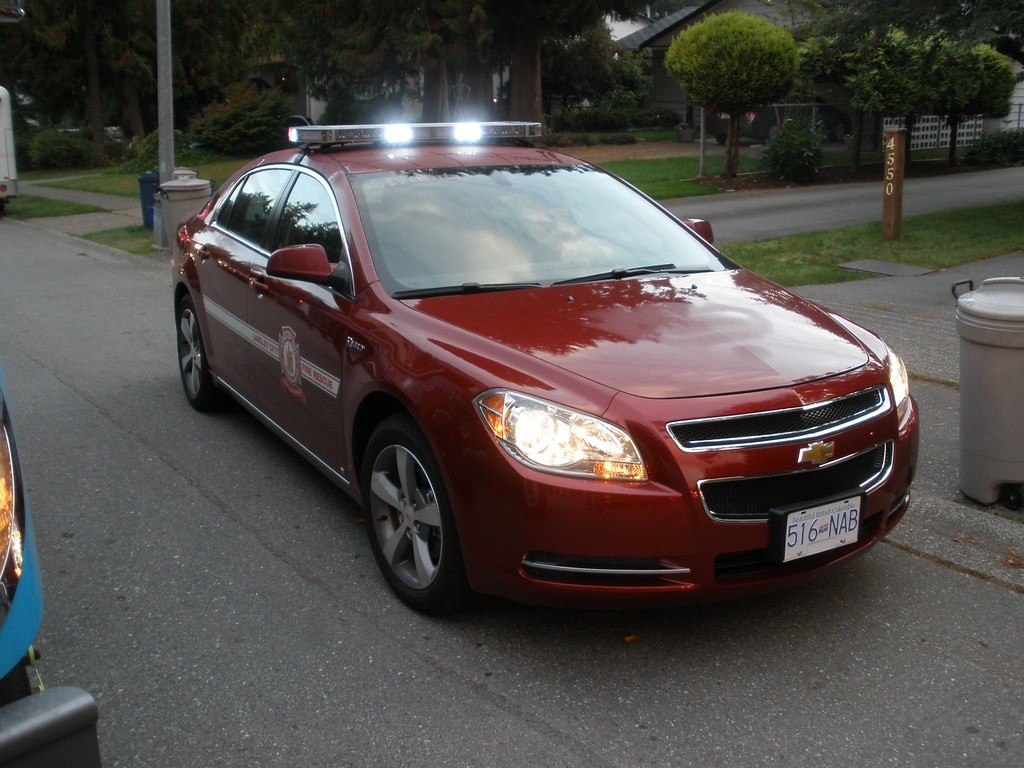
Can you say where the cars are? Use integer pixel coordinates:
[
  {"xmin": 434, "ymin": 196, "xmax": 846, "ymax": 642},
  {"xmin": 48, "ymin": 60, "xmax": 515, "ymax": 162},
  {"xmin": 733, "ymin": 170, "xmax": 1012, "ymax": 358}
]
[{"xmin": 174, "ymin": 119, "xmax": 919, "ymax": 615}]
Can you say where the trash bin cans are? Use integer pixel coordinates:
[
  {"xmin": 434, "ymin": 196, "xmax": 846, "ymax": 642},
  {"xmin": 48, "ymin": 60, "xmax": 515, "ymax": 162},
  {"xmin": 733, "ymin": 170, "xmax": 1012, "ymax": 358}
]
[
  {"xmin": 157, "ymin": 170, "xmax": 214, "ymax": 251},
  {"xmin": 137, "ymin": 170, "xmax": 158, "ymax": 229},
  {"xmin": 955, "ymin": 275, "xmax": 1024, "ymax": 504}
]
